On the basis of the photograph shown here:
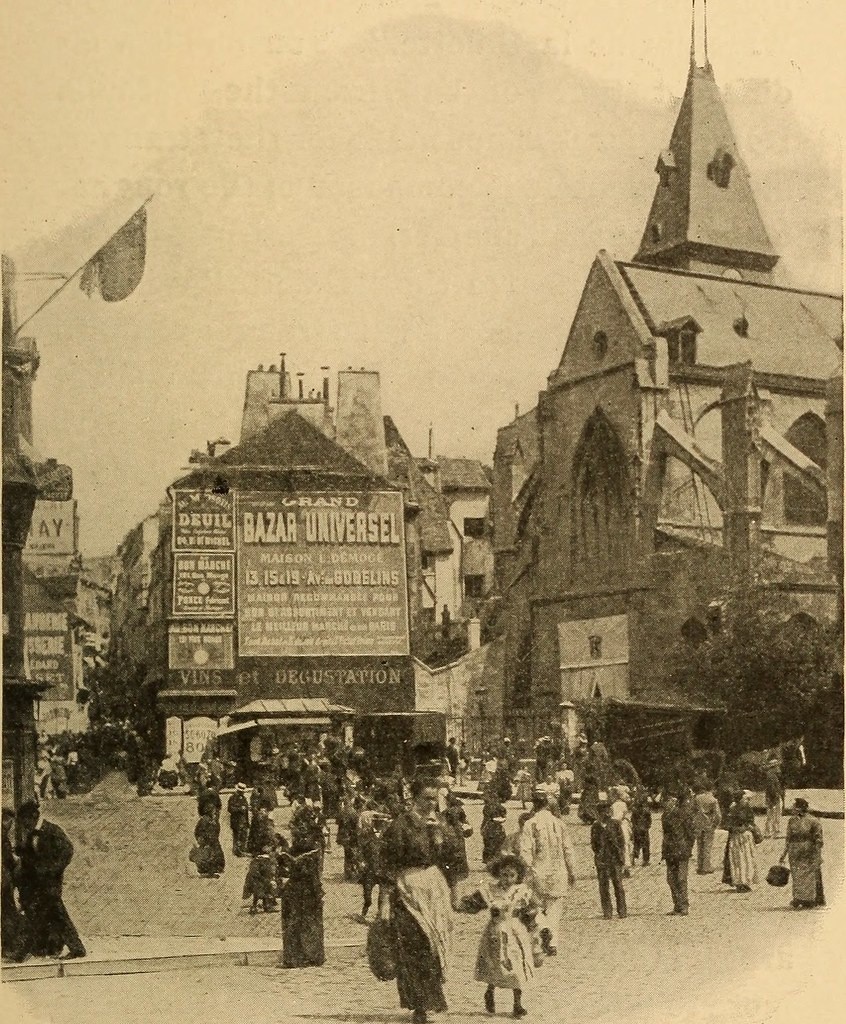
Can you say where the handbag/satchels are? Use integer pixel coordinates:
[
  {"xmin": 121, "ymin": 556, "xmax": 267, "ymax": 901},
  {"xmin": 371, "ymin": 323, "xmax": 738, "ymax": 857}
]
[
  {"xmin": 753, "ymin": 824, "xmax": 764, "ymax": 843},
  {"xmin": 367, "ymin": 918, "xmax": 397, "ymax": 982},
  {"xmin": 766, "ymin": 861, "xmax": 791, "ymax": 887}
]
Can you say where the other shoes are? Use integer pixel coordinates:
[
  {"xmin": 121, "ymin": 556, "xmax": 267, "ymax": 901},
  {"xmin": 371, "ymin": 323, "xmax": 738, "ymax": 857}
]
[
  {"xmin": 547, "ymin": 947, "xmax": 557, "ymax": 955},
  {"xmin": 667, "ymin": 909, "xmax": 687, "ymax": 916},
  {"xmin": 512, "ymin": 1005, "xmax": 528, "ymax": 1019},
  {"xmin": 6, "ymin": 951, "xmax": 24, "ymax": 963},
  {"xmin": 59, "ymin": 948, "xmax": 86, "ymax": 960},
  {"xmin": 484, "ymin": 992, "xmax": 495, "ymax": 1015},
  {"xmin": 263, "ymin": 906, "xmax": 279, "ymax": 912},
  {"xmin": 412, "ymin": 1009, "xmax": 426, "ymax": 1024},
  {"xmin": 736, "ymin": 884, "xmax": 753, "ymax": 892},
  {"xmin": 539, "ymin": 928, "xmax": 553, "ymax": 948}
]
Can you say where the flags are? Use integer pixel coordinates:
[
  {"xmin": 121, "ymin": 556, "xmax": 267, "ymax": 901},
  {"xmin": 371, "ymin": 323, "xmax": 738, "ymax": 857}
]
[{"xmin": 77, "ymin": 206, "xmax": 148, "ymax": 303}]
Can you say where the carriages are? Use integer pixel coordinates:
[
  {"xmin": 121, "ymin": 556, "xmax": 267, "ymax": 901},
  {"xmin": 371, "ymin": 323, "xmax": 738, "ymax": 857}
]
[{"xmin": 600, "ymin": 696, "xmax": 807, "ymax": 815}]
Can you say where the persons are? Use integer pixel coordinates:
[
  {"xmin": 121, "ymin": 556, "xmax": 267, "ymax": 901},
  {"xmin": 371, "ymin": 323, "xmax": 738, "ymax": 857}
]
[
  {"xmin": 2, "ymin": 800, "xmax": 86, "ymax": 964},
  {"xmin": 380, "ymin": 777, "xmax": 544, "ymax": 1024},
  {"xmin": 158, "ymin": 748, "xmax": 189, "ymax": 791},
  {"xmin": 35, "ymin": 721, "xmax": 156, "ymax": 799},
  {"xmin": 194, "ymin": 733, "xmax": 827, "ymax": 968}
]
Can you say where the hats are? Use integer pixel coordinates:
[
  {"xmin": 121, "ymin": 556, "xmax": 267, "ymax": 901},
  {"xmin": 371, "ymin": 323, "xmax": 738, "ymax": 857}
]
[
  {"xmin": 370, "ymin": 814, "xmax": 385, "ymax": 822},
  {"xmin": 16, "ymin": 802, "xmax": 40, "ymax": 817},
  {"xmin": 235, "ymin": 782, "xmax": 248, "ymax": 792},
  {"xmin": 491, "ymin": 850, "xmax": 525, "ymax": 884},
  {"xmin": 596, "ymin": 801, "xmax": 615, "ymax": 811}
]
